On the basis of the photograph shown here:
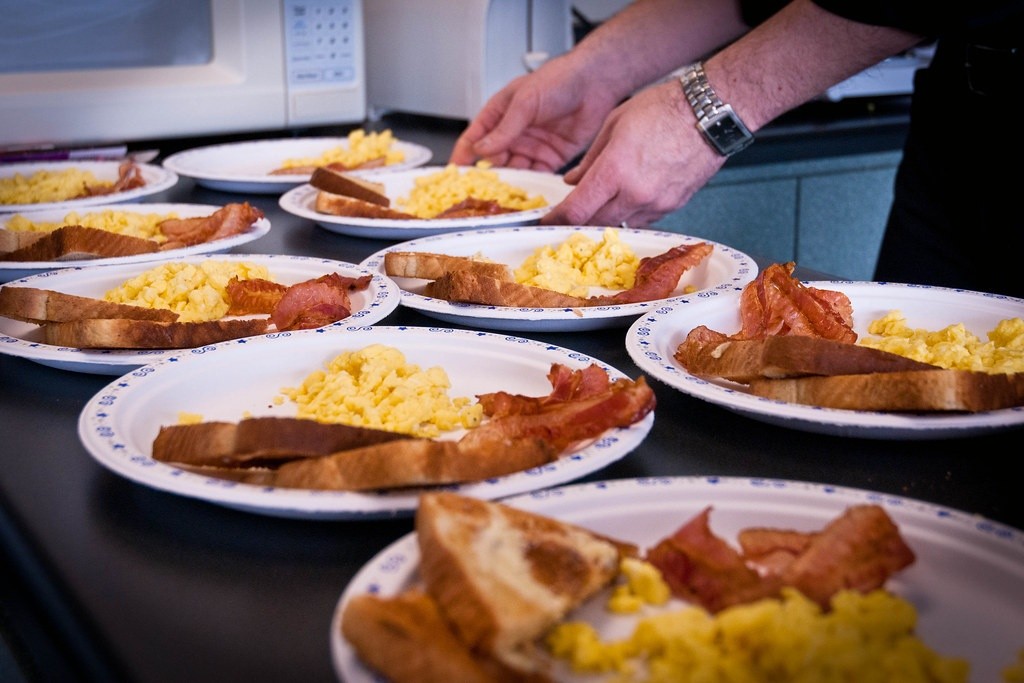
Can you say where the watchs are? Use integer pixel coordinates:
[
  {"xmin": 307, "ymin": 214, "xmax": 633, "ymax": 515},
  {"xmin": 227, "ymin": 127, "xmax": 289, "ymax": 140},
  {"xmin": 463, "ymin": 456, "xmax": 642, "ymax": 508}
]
[{"xmin": 680, "ymin": 62, "xmax": 754, "ymax": 157}]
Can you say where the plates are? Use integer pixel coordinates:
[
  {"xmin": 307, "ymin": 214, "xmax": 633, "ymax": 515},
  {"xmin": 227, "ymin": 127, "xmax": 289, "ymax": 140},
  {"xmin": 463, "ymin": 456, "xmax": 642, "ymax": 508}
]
[
  {"xmin": 0, "ymin": 253, "xmax": 402, "ymax": 376},
  {"xmin": 277, "ymin": 164, "xmax": 575, "ymax": 237},
  {"xmin": 626, "ymin": 281, "xmax": 1024, "ymax": 438},
  {"xmin": 330, "ymin": 476, "xmax": 1024, "ymax": 683},
  {"xmin": 0, "ymin": 160, "xmax": 179, "ymax": 215},
  {"xmin": 77, "ymin": 324, "xmax": 654, "ymax": 518},
  {"xmin": 0, "ymin": 203, "xmax": 271, "ymax": 283},
  {"xmin": 163, "ymin": 137, "xmax": 432, "ymax": 194},
  {"xmin": 360, "ymin": 224, "xmax": 760, "ymax": 332}
]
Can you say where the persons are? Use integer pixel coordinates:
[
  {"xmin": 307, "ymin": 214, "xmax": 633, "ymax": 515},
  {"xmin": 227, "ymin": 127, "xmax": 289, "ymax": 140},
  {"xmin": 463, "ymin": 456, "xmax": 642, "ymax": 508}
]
[{"xmin": 449, "ymin": 0, "xmax": 1024, "ymax": 301}]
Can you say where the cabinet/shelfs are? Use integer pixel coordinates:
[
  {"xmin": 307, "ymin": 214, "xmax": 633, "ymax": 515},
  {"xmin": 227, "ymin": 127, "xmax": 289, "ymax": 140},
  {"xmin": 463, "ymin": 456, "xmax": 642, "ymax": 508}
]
[{"xmin": 647, "ymin": 151, "xmax": 903, "ymax": 281}]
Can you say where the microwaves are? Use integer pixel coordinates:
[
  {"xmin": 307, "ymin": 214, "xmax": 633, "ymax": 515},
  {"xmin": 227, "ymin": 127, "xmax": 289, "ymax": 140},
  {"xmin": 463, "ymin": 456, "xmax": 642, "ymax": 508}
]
[{"xmin": 0, "ymin": 0, "xmax": 367, "ymax": 147}]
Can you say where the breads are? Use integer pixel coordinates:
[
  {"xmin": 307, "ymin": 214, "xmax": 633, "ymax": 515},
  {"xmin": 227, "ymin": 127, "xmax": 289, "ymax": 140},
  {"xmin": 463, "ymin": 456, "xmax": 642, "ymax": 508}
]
[
  {"xmin": 310, "ymin": 167, "xmax": 391, "ymax": 206},
  {"xmin": 245, "ymin": 439, "xmax": 560, "ymax": 489},
  {"xmin": 0, "ymin": 228, "xmax": 47, "ymax": 258},
  {"xmin": 316, "ymin": 179, "xmax": 420, "ymax": 219},
  {"xmin": 385, "ymin": 250, "xmax": 514, "ymax": 284},
  {"xmin": 340, "ymin": 587, "xmax": 560, "ymax": 683},
  {"xmin": 151, "ymin": 417, "xmax": 417, "ymax": 465},
  {"xmin": 423, "ymin": 270, "xmax": 613, "ymax": 307},
  {"xmin": 746, "ymin": 368, "xmax": 1024, "ymax": 412},
  {"xmin": 417, "ymin": 492, "xmax": 641, "ymax": 678},
  {"xmin": 682, "ymin": 334, "xmax": 944, "ymax": 378},
  {"xmin": 0, "ymin": 285, "xmax": 180, "ymax": 325},
  {"xmin": 41, "ymin": 319, "xmax": 268, "ymax": 348},
  {"xmin": 0, "ymin": 224, "xmax": 159, "ymax": 261}
]
